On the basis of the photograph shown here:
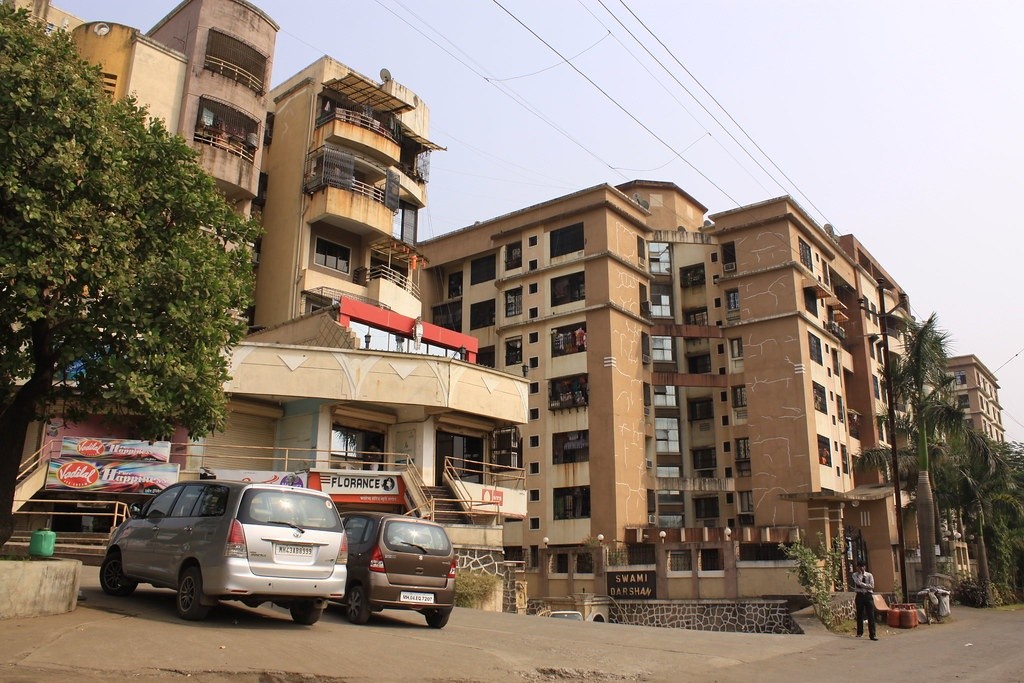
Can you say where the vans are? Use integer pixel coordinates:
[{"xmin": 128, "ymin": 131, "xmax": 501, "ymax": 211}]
[
  {"xmin": 97, "ymin": 476, "xmax": 349, "ymax": 626},
  {"xmin": 310, "ymin": 509, "xmax": 458, "ymax": 629}
]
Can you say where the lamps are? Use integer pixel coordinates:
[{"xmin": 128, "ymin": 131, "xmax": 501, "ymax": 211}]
[
  {"xmin": 543, "ymin": 537, "xmax": 549, "ymax": 548},
  {"xmin": 725, "ymin": 528, "xmax": 732, "ymax": 540},
  {"xmin": 945, "ymin": 530, "xmax": 974, "ymax": 544},
  {"xmin": 841, "ymin": 501, "xmax": 859, "ymax": 510},
  {"xmin": 598, "ymin": 535, "xmax": 604, "ymax": 542},
  {"xmin": 659, "ymin": 531, "xmax": 667, "ymax": 543}
]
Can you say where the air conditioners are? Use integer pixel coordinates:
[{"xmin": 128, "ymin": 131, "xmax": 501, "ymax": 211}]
[
  {"xmin": 638, "ymin": 257, "xmax": 645, "ymax": 268},
  {"xmin": 643, "ymin": 301, "xmax": 653, "ymax": 313},
  {"xmin": 724, "ymin": 262, "xmax": 736, "ymax": 271}
]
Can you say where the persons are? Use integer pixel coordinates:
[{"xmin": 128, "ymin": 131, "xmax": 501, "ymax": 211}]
[{"xmin": 853, "ymin": 560, "xmax": 878, "ymax": 642}]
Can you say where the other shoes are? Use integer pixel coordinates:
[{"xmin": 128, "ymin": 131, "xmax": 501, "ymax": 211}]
[
  {"xmin": 856, "ymin": 634, "xmax": 861, "ymax": 637},
  {"xmin": 870, "ymin": 637, "xmax": 878, "ymax": 641}
]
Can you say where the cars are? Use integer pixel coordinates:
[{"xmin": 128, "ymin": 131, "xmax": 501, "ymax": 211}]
[{"xmin": 547, "ymin": 610, "xmax": 583, "ymax": 621}]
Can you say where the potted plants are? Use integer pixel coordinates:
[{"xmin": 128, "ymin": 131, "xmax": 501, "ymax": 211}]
[{"xmin": 366, "ymin": 447, "xmax": 381, "ymax": 471}]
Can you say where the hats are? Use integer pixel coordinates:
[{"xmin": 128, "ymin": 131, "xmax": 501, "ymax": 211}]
[{"xmin": 857, "ymin": 559, "xmax": 866, "ymax": 567}]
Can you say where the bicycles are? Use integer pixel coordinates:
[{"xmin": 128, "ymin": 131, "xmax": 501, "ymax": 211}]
[{"xmin": 922, "ymin": 585, "xmax": 950, "ymax": 626}]
